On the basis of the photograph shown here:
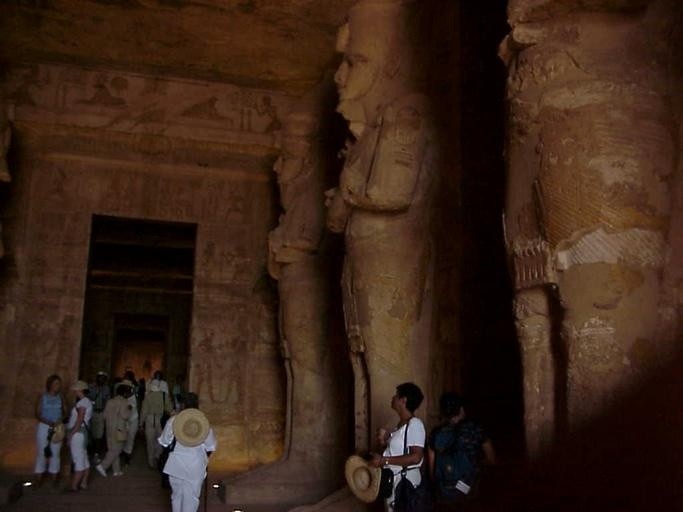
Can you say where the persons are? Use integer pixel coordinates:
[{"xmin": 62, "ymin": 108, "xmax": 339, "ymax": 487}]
[
  {"xmin": 322, "ymin": 4, "xmax": 441, "ymax": 500},
  {"xmin": 258, "ymin": 111, "xmax": 335, "ymax": 466},
  {"xmin": 356, "ymin": 384, "xmax": 499, "ymax": 512},
  {"xmin": 31, "ymin": 368, "xmax": 219, "ymax": 512},
  {"xmin": 493, "ymin": 2, "xmax": 661, "ymax": 475}
]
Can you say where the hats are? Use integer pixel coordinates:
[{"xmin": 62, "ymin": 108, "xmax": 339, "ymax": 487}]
[
  {"xmin": 172, "ymin": 407, "xmax": 210, "ymax": 447},
  {"xmin": 117, "ymin": 379, "xmax": 135, "ymax": 389},
  {"xmin": 345, "ymin": 454, "xmax": 383, "ymax": 504},
  {"xmin": 70, "ymin": 380, "xmax": 89, "ymax": 390}
]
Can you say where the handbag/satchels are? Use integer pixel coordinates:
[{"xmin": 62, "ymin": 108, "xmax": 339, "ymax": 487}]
[
  {"xmin": 379, "ymin": 466, "xmax": 394, "ymax": 498},
  {"xmin": 160, "ymin": 413, "xmax": 170, "ymax": 430},
  {"xmin": 392, "ymin": 476, "xmax": 426, "ymax": 512},
  {"xmin": 44, "ymin": 446, "xmax": 53, "ymax": 458},
  {"xmin": 116, "ymin": 428, "xmax": 128, "ymax": 442}
]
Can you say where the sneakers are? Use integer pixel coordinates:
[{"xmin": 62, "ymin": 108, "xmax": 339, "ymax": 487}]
[{"xmin": 95, "ymin": 464, "xmax": 125, "ymax": 477}]
